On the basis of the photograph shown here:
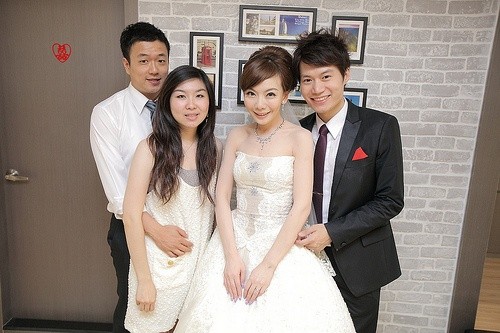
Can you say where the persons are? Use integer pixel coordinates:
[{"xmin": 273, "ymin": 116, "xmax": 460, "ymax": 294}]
[
  {"xmin": 214, "ymin": 45, "xmax": 314, "ymax": 333},
  {"xmin": 122, "ymin": 64, "xmax": 225, "ymax": 333},
  {"xmin": 90, "ymin": 21, "xmax": 194, "ymax": 333},
  {"xmin": 294, "ymin": 32, "xmax": 404, "ymax": 332}
]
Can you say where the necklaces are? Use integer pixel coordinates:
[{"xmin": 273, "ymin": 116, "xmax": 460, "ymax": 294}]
[{"xmin": 254, "ymin": 117, "xmax": 286, "ymax": 150}]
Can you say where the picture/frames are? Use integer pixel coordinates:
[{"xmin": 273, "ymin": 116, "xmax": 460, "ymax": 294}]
[
  {"xmin": 332, "ymin": 16, "xmax": 368, "ymax": 64},
  {"xmin": 239, "ymin": 5, "xmax": 317, "ymax": 45},
  {"xmin": 343, "ymin": 88, "xmax": 367, "ymax": 108},
  {"xmin": 189, "ymin": 32, "xmax": 224, "ymax": 110},
  {"xmin": 237, "ymin": 60, "xmax": 308, "ymax": 104}
]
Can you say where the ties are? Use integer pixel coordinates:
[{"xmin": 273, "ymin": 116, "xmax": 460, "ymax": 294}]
[
  {"xmin": 312, "ymin": 124, "xmax": 330, "ymax": 224},
  {"xmin": 145, "ymin": 101, "xmax": 157, "ymax": 120}
]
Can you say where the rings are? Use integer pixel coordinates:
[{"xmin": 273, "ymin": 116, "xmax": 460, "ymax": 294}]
[{"xmin": 255, "ymin": 287, "xmax": 260, "ymax": 291}]
